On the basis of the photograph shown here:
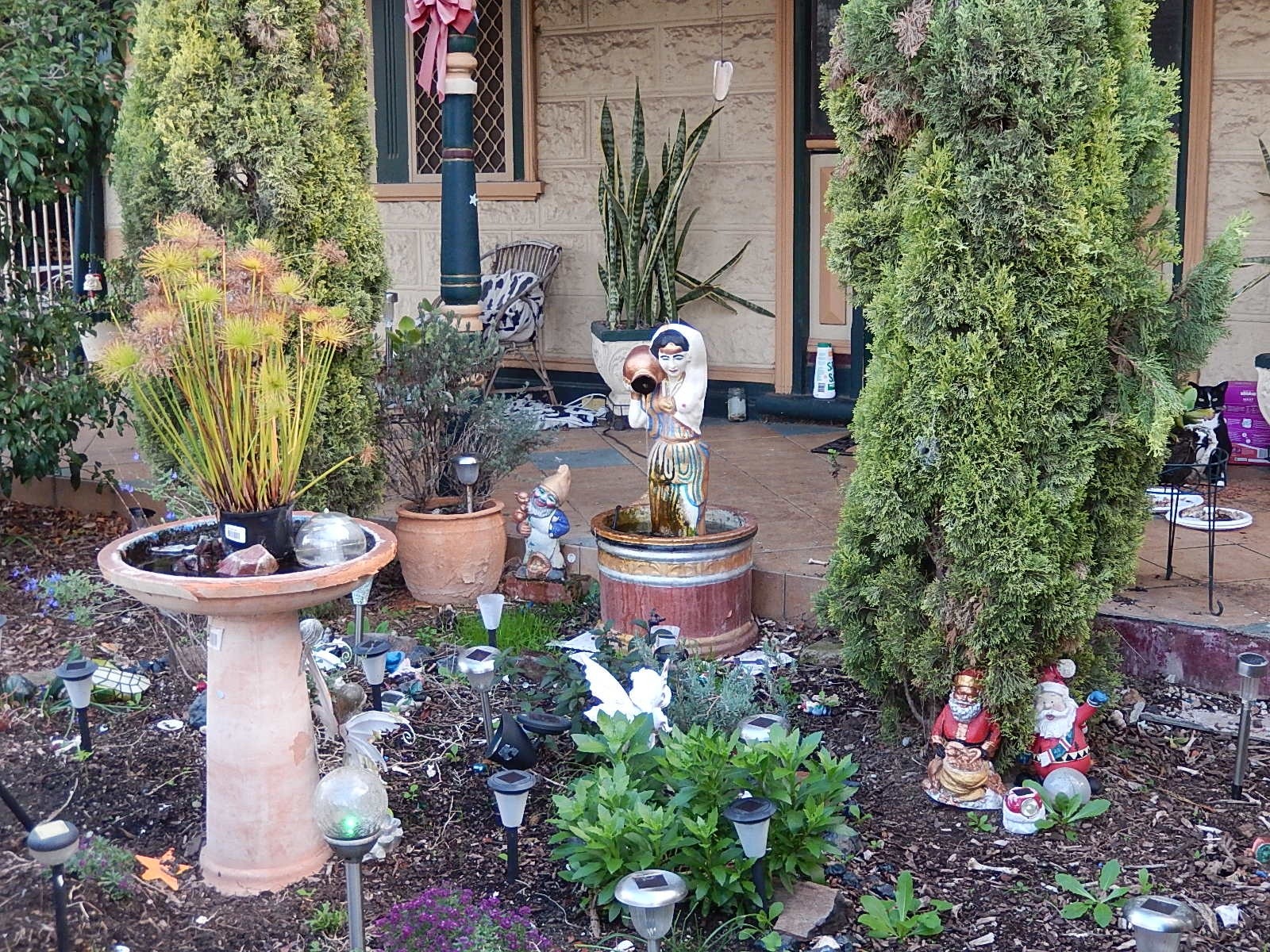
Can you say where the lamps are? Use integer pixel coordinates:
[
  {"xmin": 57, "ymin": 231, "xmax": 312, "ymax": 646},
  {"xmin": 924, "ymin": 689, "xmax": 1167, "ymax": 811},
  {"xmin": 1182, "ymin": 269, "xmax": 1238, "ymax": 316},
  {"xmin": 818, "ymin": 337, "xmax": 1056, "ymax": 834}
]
[
  {"xmin": 1124, "ymin": 896, "xmax": 1197, "ymax": 952},
  {"xmin": 488, "ymin": 770, "xmax": 537, "ymax": 882},
  {"xmin": 1231, "ymin": 652, "xmax": 1269, "ymax": 800},
  {"xmin": 56, "ymin": 658, "xmax": 98, "ymax": 752},
  {"xmin": 449, "ymin": 455, "xmax": 483, "ymax": 515},
  {"xmin": 724, "ymin": 798, "xmax": 777, "ymax": 896},
  {"xmin": 354, "ymin": 641, "xmax": 391, "ymax": 712},
  {"xmin": 312, "ymin": 766, "xmax": 389, "ymax": 952},
  {"xmin": 614, "ymin": 868, "xmax": 689, "ymax": 952},
  {"xmin": 353, "ymin": 576, "xmax": 374, "ymax": 644},
  {"xmin": 477, "ymin": 593, "xmax": 505, "ymax": 648},
  {"xmin": 24, "ymin": 820, "xmax": 80, "ymax": 952}
]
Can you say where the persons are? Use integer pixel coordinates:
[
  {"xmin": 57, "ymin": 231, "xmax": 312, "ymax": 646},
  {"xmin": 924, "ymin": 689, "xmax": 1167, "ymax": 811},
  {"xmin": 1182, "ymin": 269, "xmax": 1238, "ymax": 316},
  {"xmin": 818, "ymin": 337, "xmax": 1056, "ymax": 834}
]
[
  {"xmin": 622, "ymin": 323, "xmax": 710, "ymax": 536},
  {"xmin": 515, "ymin": 463, "xmax": 571, "ymax": 580},
  {"xmin": 1014, "ymin": 658, "xmax": 1108, "ymax": 795},
  {"xmin": 931, "ymin": 669, "xmax": 1001, "ymax": 770}
]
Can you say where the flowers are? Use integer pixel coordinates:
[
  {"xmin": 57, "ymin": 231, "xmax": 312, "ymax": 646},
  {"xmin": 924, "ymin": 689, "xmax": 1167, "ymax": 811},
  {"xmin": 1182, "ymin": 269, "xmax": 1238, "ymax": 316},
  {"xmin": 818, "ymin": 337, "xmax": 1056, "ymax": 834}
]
[{"xmin": 92, "ymin": 211, "xmax": 371, "ymax": 512}]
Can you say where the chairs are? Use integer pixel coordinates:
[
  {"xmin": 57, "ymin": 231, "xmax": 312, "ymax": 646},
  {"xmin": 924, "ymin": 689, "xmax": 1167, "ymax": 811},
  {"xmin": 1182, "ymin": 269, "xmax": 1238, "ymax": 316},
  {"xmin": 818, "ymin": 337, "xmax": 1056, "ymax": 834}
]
[{"xmin": 432, "ymin": 240, "xmax": 563, "ymax": 409}]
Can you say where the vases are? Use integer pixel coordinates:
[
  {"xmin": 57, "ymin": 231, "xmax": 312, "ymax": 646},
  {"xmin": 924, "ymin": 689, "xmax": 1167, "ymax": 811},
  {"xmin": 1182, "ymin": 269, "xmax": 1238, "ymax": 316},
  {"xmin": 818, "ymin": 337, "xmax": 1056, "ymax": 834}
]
[{"xmin": 215, "ymin": 508, "xmax": 291, "ymax": 562}]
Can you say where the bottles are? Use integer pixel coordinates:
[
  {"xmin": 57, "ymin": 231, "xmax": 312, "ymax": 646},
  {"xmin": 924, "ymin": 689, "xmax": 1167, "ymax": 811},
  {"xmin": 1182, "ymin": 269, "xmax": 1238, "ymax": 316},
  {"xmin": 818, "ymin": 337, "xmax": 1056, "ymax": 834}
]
[
  {"xmin": 728, "ymin": 388, "xmax": 746, "ymax": 422},
  {"xmin": 623, "ymin": 345, "xmax": 664, "ymax": 395},
  {"xmin": 812, "ymin": 343, "xmax": 836, "ymax": 398}
]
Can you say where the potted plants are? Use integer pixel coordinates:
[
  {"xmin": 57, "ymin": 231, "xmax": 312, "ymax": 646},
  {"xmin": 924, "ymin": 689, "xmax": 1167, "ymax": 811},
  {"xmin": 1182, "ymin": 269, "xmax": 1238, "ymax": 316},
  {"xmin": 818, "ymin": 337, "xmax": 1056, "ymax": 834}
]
[
  {"xmin": 576, "ymin": 79, "xmax": 778, "ymax": 416},
  {"xmin": 368, "ymin": 302, "xmax": 565, "ymax": 608}
]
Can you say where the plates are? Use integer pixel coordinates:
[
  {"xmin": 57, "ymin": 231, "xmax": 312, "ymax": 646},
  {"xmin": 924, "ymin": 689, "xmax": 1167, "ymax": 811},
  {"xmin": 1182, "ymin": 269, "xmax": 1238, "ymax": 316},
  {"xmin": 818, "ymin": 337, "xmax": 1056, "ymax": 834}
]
[{"xmin": 1146, "ymin": 488, "xmax": 1253, "ymax": 529}]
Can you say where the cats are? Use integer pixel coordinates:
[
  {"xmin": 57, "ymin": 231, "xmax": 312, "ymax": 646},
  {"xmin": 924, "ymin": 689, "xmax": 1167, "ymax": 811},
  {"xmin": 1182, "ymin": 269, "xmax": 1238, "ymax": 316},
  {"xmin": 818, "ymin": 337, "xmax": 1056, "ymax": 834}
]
[{"xmin": 1186, "ymin": 379, "xmax": 1233, "ymax": 481}]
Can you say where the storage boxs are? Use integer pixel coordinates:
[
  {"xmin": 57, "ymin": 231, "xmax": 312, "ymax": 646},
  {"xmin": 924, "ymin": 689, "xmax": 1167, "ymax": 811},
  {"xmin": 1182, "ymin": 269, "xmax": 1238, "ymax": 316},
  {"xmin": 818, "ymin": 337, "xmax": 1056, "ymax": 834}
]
[{"xmin": 1223, "ymin": 381, "xmax": 1270, "ymax": 465}]
